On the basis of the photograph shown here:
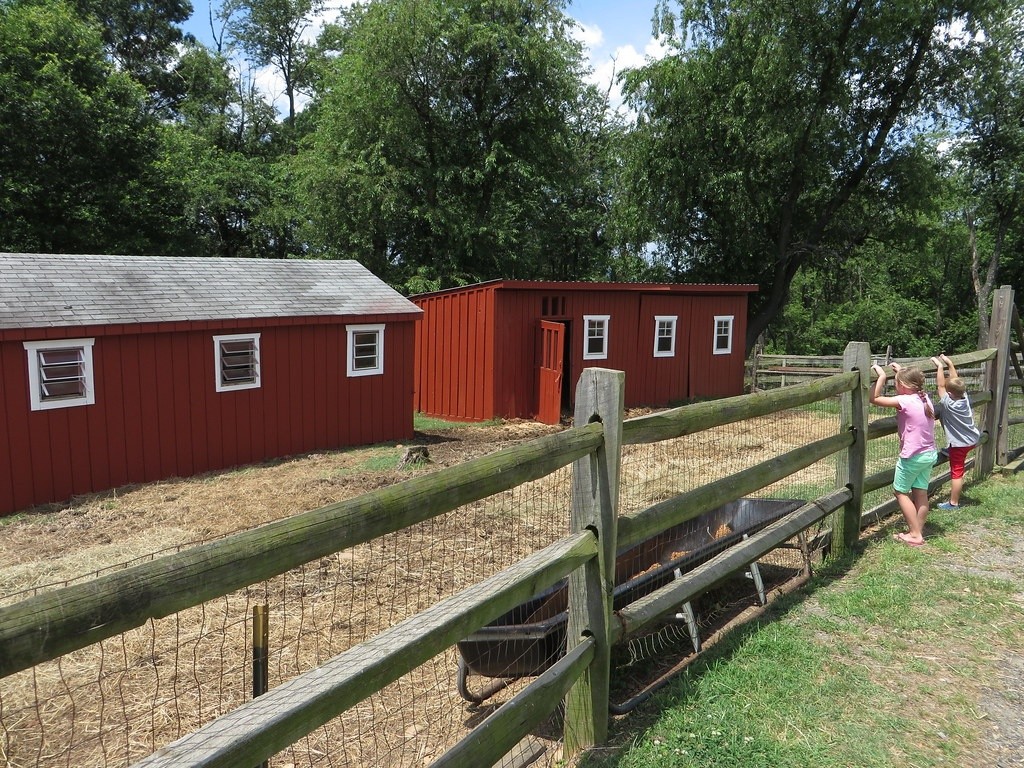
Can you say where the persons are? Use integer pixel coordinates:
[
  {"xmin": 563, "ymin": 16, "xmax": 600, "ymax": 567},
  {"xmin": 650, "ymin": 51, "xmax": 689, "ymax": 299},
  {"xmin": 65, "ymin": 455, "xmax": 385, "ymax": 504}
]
[
  {"xmin": 868, "ymin": 363, "xmax": 938, "ymax": 546},
  {"xmin": 930, "ymin": 354, "xmax": 980, "ymax": 511}
]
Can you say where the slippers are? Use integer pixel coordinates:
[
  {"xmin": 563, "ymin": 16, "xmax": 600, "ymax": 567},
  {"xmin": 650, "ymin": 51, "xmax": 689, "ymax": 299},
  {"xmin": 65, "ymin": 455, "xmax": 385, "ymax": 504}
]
[{"xmin": 894, "ymin": 533, "xmax": 918, "ymax": 546}]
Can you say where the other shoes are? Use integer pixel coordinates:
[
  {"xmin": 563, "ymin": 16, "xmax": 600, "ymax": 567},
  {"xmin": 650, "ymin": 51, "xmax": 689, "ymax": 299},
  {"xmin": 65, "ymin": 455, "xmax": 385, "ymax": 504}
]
[{"xmin": 937, "ymin": 498, "xmax": 960, "ymax": 510}]
